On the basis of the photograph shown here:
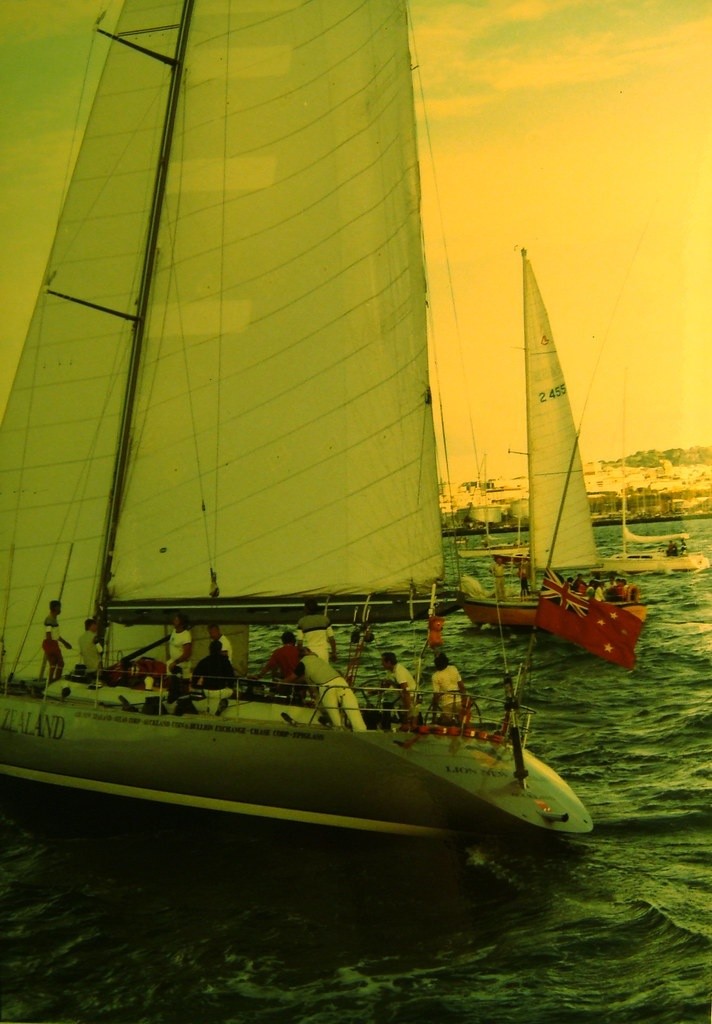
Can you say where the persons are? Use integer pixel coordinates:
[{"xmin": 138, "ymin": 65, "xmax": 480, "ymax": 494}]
[
  {"xmin": 206, "ymin": 624, "xmax": 232, "ymax": 663},
  {"xmin": 295, "ymin": 598, "xmax": 336, "ymax": 665},
  {"xmin": 78, "ymin": 619, "xmax": 104, "ymax": 674},
  {"xmin": 191, "ymin": 641, "xmax": 237, "ymax": 718},
  {"xmin": 274, "ymin": 648, "xmax": 367, "ymax": 733},
  {"xmin": 518, "ymin": 564, "xmax": 530, "ymax": 598},
  {"xmin": 248, "ymin": 631, "xmax": 317, "ymax": 701},
  {"xmin": 380, "ymin": 652, "xmax": 421, "ymax": 721},
  {"xmin": 488, "ymin": 558, "xmax": 509, "ymax": 603},
  {"xmin": 666, "ymin": 538, "xmax": 688, "ymax": 557},
  {"xmin": 431, "ymin": 653, "xmax": 470, "ymax": 728},
  {"xmin": 565, "ymin": 571, "xmax": 629, "ymax": 602},
  {"xmin": 43, "ymin": 600, "xmax": 72, "ymax": 685},
  {"xmin": 166, "ymin": 614, "xmax": 193, "ymax": 676}
]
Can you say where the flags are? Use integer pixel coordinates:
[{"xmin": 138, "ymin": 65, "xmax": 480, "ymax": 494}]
[{"xmin": 534, "ymin": 567, "xmax": 644, "ymax": 670}]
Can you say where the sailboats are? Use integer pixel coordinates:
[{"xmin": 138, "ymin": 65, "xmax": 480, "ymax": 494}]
[
  {"xmin": 461, "ymin": 249, "xmax": 649, "ymax": 628},
  {"xmin": 1, "ymin": 1, "xmax": 598, "ymax": 851},
  {"xmin": 457, "ymin": 455, "xmax": 529, "ymax": 560},
  {"xmin": 493, "ymin": 367, "xmax": 711, "ymax": 573}
]
